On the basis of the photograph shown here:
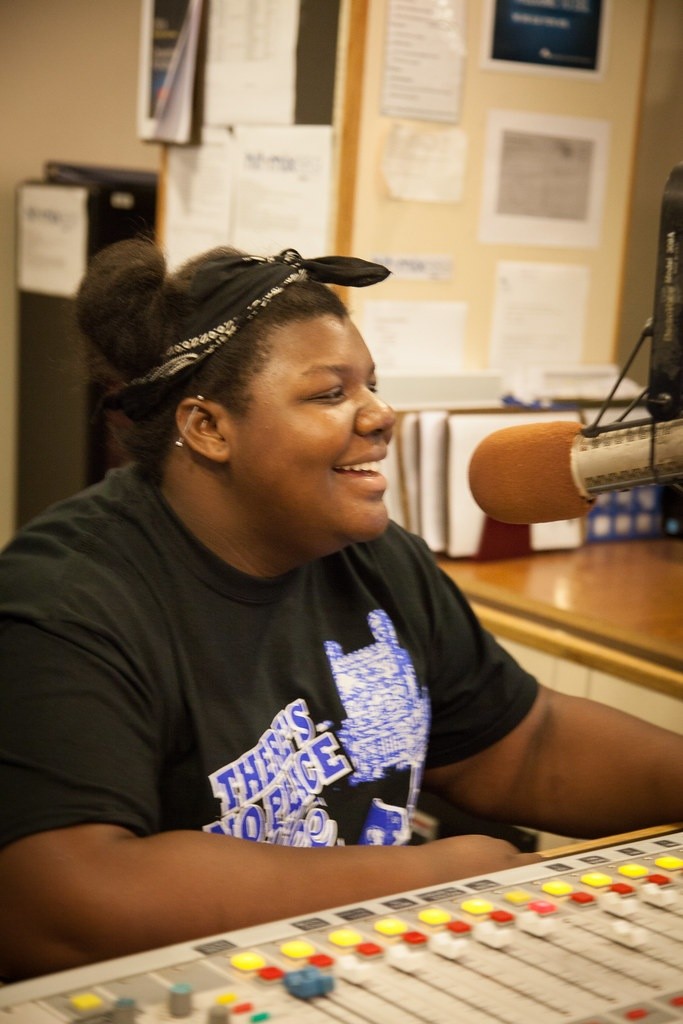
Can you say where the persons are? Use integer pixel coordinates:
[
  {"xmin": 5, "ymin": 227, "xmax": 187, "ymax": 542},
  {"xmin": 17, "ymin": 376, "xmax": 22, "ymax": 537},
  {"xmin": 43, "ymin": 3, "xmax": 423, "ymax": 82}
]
[{"xmin": 0, "ymin": 236, "xmax": 683, "ymax": 975}]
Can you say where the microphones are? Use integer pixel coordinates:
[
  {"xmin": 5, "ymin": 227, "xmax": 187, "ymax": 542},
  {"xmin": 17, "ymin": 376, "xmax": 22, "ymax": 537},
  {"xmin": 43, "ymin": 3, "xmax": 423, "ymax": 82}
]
[{"xmin": 468, "ymin": 419, "xmax": 682, "ymax": 524}]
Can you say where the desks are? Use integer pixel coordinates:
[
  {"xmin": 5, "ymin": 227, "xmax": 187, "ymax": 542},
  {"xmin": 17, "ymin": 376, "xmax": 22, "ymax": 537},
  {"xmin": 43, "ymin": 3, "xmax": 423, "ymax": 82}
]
[{"xmin": 444, "ymin": 537, "xmax": 680, "ymax": 738}]
